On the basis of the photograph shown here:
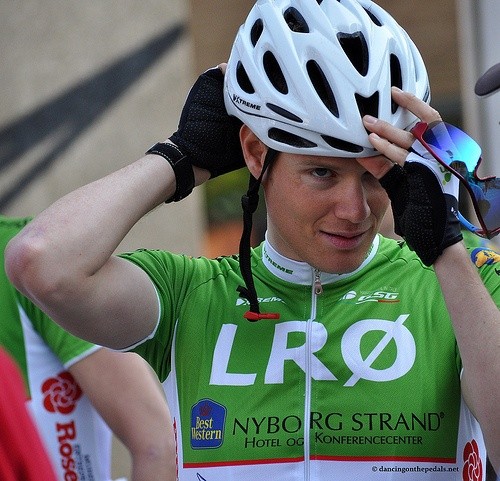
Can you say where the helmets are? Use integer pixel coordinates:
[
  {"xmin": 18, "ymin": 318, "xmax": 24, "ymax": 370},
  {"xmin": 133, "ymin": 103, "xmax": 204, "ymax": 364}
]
[{"xmin": 225, "ymin": 0, "xmax": 429, "ymax": 158}]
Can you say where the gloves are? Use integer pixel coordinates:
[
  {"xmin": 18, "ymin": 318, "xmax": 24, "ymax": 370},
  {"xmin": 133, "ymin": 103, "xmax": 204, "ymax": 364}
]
[{"xmin": 171, "ymin": 64, "xmax": 259, "ymax": 180}]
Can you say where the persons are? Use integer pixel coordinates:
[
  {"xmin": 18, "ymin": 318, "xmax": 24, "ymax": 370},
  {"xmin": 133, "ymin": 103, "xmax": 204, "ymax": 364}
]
[
  {"xmin": 0, "ymin": 214, "xmax": 177, "ymax": 481},
  {"xmin": 4, "ymin": 0, "xmax": 500, "ymax": 481}
]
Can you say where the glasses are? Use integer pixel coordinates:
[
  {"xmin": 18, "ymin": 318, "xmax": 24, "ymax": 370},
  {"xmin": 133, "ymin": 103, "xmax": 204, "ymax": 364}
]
[{"xmin": 406, "ymin": 121, "xmax": 500, "ymax": 239}]
